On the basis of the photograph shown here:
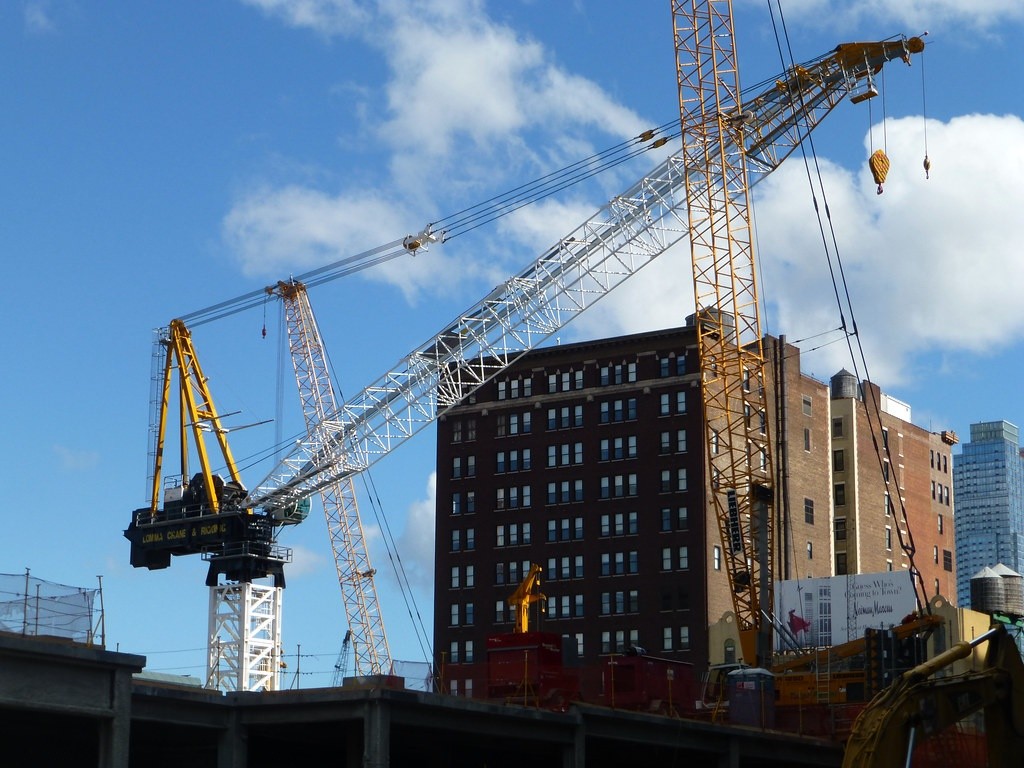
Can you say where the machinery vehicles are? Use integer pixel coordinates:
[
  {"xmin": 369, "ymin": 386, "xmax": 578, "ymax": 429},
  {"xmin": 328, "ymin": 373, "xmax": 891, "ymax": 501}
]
[{"xmin": 343, "ymin": 1, "xmax": 1024, "ymax": 768}]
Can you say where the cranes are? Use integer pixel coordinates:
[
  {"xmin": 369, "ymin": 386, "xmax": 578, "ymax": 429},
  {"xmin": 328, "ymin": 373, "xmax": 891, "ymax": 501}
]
[
  {"xmin": 261, "ymin": 278, "xmax": 434, "ymax": 677},
  {"xmin": 122, "ymin": 29, "xmax": 934, "ymax": 694}
]
